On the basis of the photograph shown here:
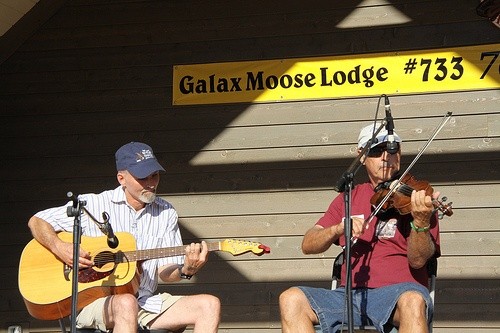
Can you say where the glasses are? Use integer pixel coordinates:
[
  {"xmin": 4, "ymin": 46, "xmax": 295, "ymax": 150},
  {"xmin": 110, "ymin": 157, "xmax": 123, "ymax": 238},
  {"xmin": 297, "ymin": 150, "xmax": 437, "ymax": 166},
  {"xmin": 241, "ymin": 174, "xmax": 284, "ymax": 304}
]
[{"xmin": 368, "ymin": 142, "xmax": 400, "ymax": 157}]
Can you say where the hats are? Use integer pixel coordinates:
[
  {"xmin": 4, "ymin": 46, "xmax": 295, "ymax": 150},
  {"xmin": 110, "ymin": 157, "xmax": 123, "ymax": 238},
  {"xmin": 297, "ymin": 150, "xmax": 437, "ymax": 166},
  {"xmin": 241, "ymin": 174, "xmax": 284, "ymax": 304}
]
[
  {"xmin": 114, "ymin": 142, "xmax": 166, "ymax": 179},
  {"xmin": 358, "ymin": 123, "xmax": 402, "ymax": 149}
]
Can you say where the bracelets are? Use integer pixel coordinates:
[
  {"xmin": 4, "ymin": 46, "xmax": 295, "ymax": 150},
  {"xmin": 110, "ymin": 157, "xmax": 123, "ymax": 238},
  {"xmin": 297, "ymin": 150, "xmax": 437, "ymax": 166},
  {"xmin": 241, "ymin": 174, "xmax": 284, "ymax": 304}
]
[
  {"xmin": 410, "ymin": 222, "xmax": 430, "ymax": 232},
  {"xmin": 334, "ymin": 224, "xmax": 340, "ymax": 236}
]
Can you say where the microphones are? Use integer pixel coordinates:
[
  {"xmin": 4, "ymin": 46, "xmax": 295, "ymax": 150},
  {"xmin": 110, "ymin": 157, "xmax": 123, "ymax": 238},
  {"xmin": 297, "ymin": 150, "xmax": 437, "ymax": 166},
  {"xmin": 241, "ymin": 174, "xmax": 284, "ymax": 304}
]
[
  {"xmin": 102, "ymin": 213, "xmax": 118, "ymax": 248},
  {"xmin": 385, "ymin": 95, "xmax": 400, "ymax": 155}
]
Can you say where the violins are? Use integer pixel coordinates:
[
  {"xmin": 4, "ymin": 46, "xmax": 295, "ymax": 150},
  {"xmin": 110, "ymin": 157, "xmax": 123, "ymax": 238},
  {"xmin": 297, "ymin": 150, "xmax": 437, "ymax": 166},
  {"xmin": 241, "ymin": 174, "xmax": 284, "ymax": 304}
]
[{"xmin": 369, "ymin": 169, "xmax": 455, "ymax": 220}]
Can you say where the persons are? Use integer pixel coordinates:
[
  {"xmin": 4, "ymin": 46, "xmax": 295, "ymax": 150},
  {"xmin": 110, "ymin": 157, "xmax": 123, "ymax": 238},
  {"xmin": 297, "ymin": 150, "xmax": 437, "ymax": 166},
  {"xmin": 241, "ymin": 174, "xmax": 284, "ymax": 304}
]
[
  {"xmin": 278, "ymin": 121, "xmax": 442, "ymax": 333},
  {"xmin": 28, "ymin": 140, "xmax": 221, "ymax": 333}
]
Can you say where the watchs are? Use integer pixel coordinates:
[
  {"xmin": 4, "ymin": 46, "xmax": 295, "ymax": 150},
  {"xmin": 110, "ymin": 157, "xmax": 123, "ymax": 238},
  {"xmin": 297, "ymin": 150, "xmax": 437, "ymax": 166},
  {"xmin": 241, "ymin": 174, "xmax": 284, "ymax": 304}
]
[{"xmin": 177, "ymin": 264, "xmax": 196, "ymax": 280}]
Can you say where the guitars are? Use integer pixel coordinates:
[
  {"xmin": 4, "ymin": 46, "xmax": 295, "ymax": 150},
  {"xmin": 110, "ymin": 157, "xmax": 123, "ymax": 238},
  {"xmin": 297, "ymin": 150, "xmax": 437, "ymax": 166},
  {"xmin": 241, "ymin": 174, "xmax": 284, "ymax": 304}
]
[{"xmin": 17, "ymin": 230, "xmax": 271, "ymax": 322}]
